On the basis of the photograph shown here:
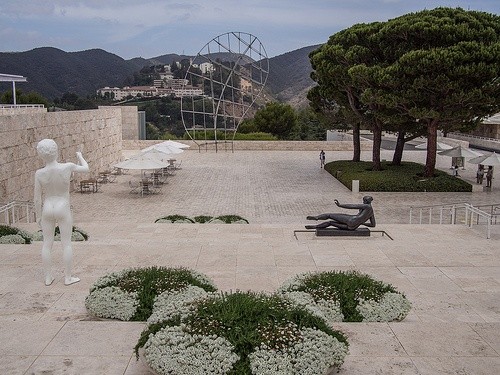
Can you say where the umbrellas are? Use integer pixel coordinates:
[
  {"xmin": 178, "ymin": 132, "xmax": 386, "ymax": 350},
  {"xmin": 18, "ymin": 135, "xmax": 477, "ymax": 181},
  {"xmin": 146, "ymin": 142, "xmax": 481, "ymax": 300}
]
[{"xmin": 113, "ymin": 138, "xmax": 191, "ymax": 194}]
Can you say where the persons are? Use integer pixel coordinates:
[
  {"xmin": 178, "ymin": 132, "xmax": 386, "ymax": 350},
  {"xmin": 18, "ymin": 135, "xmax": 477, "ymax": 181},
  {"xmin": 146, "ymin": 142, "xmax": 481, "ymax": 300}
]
[
  {"xmin": 319, "ymin": 150, "xmax": 325, "ymax": 168},
  {"xmin": 477, "ymin": 165, "xmax": 493, "ymax": 188},
  {"xmin": 33, "ymin": 139, "xmax": 90, "ymax": 286},
  {"xmin": 305, "ymin": 195, "xmax": 376, "ymax": 230}
]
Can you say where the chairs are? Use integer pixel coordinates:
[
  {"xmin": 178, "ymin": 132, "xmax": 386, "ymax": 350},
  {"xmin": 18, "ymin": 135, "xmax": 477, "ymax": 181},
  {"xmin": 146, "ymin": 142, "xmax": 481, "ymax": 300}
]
[{"xmin": 128, "ymin": 180, "xmax": 139, "ymax": 194}]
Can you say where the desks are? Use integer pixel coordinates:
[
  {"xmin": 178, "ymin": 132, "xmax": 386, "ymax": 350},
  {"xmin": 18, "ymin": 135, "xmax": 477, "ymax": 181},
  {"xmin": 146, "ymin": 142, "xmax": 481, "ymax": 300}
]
[{"xmin": 80, "ymin": 180, "xmax": 97, "ymax": 193}]
